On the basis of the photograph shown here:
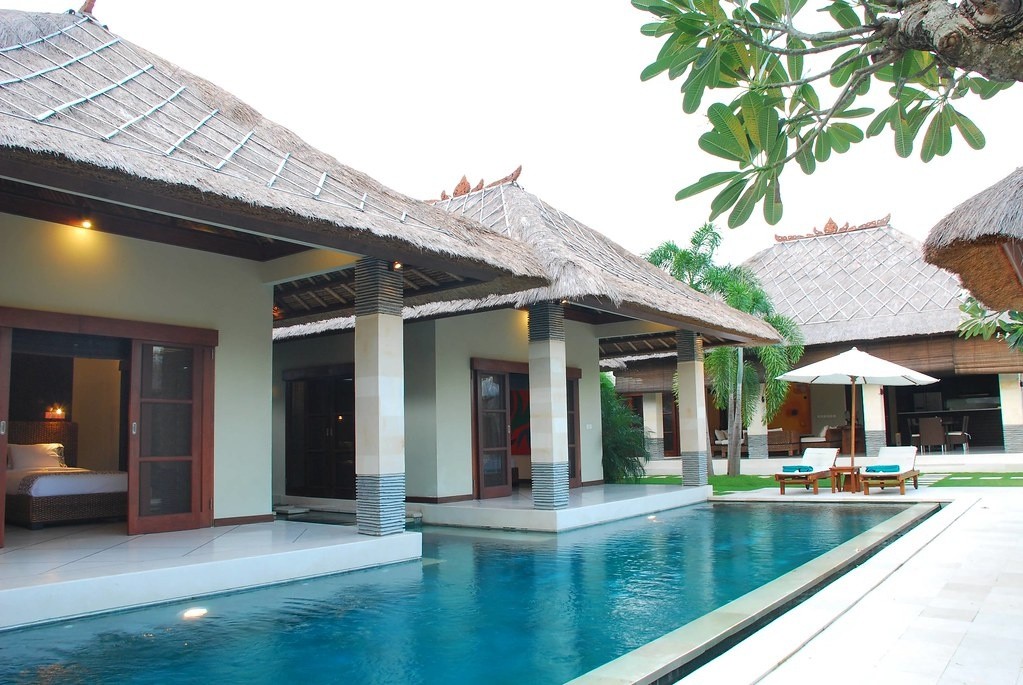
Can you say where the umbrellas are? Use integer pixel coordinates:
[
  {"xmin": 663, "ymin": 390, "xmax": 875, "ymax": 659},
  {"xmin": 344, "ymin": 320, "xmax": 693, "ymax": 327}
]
[{"xmin": 774, "ymin": 346, "xmax": 941, "ymax": 466}]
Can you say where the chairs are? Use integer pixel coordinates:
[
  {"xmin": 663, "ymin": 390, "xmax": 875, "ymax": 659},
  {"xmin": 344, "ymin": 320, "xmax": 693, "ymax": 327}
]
[{"xmin": 714, "ymin": 415, "xmax": 970, "ymax": 494}]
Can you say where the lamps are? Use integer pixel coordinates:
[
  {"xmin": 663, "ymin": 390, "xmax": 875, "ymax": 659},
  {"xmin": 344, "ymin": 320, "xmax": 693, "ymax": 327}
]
[{"xmin": 44, "ymin": 402, "xmax": 65, "ymax": 419}]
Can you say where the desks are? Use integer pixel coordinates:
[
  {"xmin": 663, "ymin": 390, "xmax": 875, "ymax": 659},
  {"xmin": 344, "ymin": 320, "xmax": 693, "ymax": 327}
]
[
  {"xmin": 943, "ymin": 421, "xmax": 959, "ymax": 451},
  {"xmin": 829, "ymin": 467, "xmax": 861, "ymax": 494}
]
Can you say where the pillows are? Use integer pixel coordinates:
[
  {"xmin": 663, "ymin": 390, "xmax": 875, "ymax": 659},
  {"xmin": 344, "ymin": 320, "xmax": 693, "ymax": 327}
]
[{"xmin": 7, "ymin": 443, "xmax": 68, "ymax": 469}]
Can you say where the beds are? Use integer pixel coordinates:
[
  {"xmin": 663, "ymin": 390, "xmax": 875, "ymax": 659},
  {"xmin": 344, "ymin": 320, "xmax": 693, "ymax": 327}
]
[{"xmin": 6, "ymin": 421, "xmax": 128, "ymax": 530}]
